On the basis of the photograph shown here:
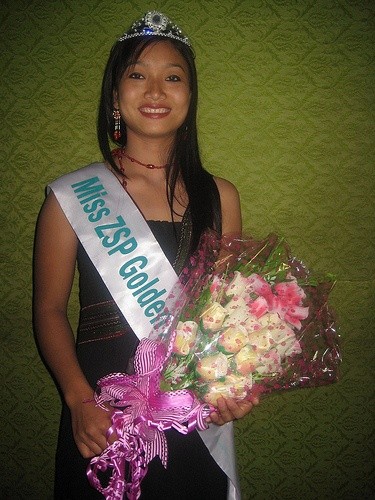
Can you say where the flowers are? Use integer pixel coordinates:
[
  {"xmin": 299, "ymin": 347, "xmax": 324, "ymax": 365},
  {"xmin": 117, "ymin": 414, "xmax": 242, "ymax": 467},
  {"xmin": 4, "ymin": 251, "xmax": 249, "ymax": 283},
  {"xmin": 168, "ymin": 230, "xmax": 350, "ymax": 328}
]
[{"xmin": 166, "ymin": 268, "xmax": 308, "ymax": 409}]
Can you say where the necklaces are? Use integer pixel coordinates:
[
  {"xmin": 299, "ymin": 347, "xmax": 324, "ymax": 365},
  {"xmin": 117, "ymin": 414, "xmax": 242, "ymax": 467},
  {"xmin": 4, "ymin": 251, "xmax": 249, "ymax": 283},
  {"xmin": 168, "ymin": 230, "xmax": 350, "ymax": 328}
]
[{"xmin": 109, "ymin": 146, "xmax": 176, "ymax": 191}]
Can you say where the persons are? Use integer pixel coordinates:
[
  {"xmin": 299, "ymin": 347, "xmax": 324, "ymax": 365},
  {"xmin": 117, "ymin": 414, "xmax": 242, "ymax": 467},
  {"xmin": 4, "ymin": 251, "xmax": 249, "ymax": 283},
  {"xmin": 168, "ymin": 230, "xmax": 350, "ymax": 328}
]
[{"xmin": 33, "ymin": 11, "xmax": 262, "ymax": 500}]
